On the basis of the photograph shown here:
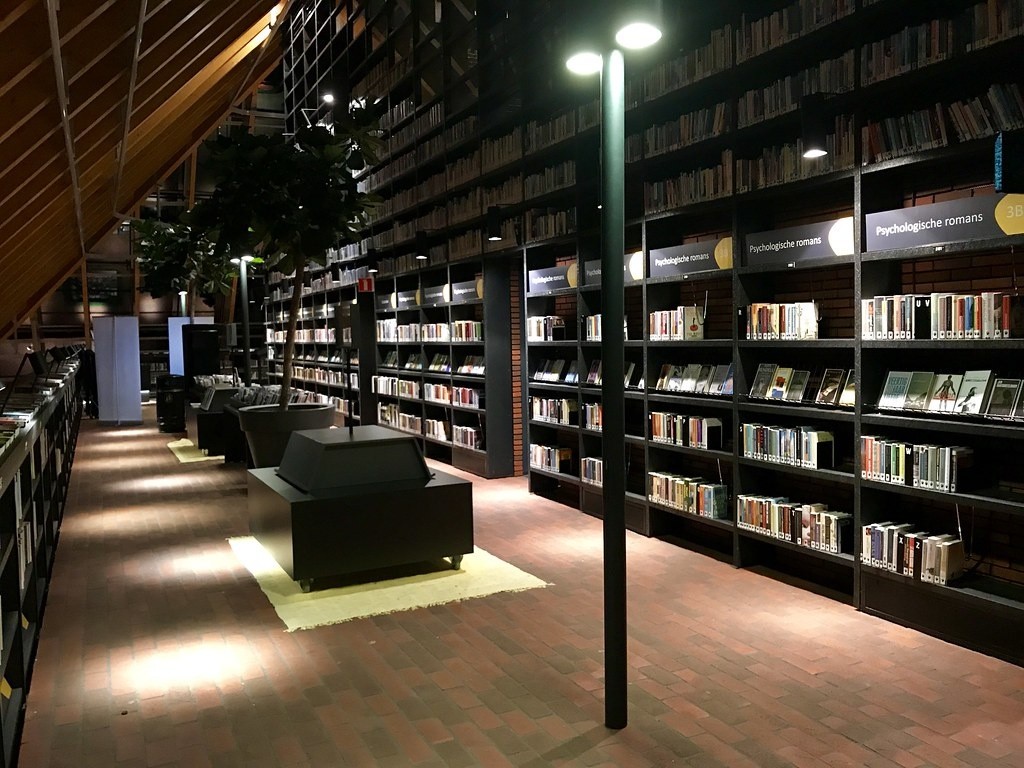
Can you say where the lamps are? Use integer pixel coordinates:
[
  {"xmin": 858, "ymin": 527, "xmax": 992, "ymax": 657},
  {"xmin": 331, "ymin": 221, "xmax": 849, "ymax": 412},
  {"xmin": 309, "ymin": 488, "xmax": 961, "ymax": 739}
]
[
  {"xmin": 263, "ymin": 284, "xmax": 279, "ymax": 299},
  {"xmin": 799, "ymin": 92, "xmax": 854, "ymax": 161},
  {"xmin": 45, "ymin": 346, "xmax": 70, "ymax": 382},
  {"xmin": 367, "ymin": 247, "xmax": 386, "ymax": 273},
  {"xmin": 304, "ymin": 271, "xmax": 319, "ymax": 290},
  {"xmin": 32, "ymin": 346, "xmax": 64, "ymax": 390},
  {"xmin": 415, "ymin": 229, "xmax": 437, "ymax": 260},
  {"xmin": 55, "ymin": 346, "xmax": 76, "ymax": 374},
  {"xmin": 281, "ymin": 278, "xmax": 293, "ymax": 294},
  {"xmin": 0, "ymin": 352, "xmax": 50, "ymax": 415},
  {"xmin": 331, "ymin": 261, "xmax": 349, "ymax": 283},
  {"xmin": 487, "ymin": 204, "xmax": 514, "ymax": 241}
]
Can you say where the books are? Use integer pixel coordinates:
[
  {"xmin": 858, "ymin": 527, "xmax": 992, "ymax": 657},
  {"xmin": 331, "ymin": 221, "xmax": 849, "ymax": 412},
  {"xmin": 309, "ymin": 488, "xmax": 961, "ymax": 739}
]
[
  {"xmin": 0, "ymin": 355, "xmax": 81, "ymax": 671},
  {"xmin": 529, "ymin": 443, "xmax": 572, "ymax": 474},
  {"xmin": 272, "ymin": 363, "xmax": 485, "ymax": 450},
  {"xmin": 580, "ymin": 402, "xmax": 603, "ymax": 431},
  {"xmin": 529, "ymin": 396, "xmax": 579, "ymax": 425},
  {"xmin": 264, "ymin": 0, "xmax": 1024, "ymax": 304},
  {"xmin": 529, "ymin": 356, "xmax": 578, "ymax": 384},
  {"xmin": 586, "ymin": 356, "xmax": 644, "ymax": 389},
  {"xmin": 525, "ymin": 315, "xmax": 566, "ymax": 341},
  {"xmin": 266, "ymin": 319, "xmax": 483, "ymax": 345},
  {"xmin": 234, "ymin": 385, "xmax": 314, "ymax": 407},
  {"xmin": 580, "ymin": 456, "xmax": 604, "ymax": 490},
  {"xmin": 267, "ymin": 344, "xmax": 486, "ymax": 376},
  {"xmin": 648, "ymin": 292, "xmax": 1024, "ymax": 587},
  {"xmin": 581, "ymin": 313, "xmax": 629, "ymax": 341}
]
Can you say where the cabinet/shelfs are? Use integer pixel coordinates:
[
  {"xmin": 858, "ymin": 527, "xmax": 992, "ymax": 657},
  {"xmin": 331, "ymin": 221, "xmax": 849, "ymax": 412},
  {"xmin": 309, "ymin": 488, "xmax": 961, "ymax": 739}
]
[
  {"xmin": 0, "ymin": 343, "xmax": 170, "ymax": 768},
  {"xmin": 215, "ymin": 0, "xmax": 1024, "ymax": 670}
]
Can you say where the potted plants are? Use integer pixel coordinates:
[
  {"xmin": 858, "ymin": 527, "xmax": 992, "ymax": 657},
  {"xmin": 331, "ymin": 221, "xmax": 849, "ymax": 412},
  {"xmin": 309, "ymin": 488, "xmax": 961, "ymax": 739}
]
[{"xmin": 206, "ymin": 90, "xmax": 390, "ymax": 469}]
